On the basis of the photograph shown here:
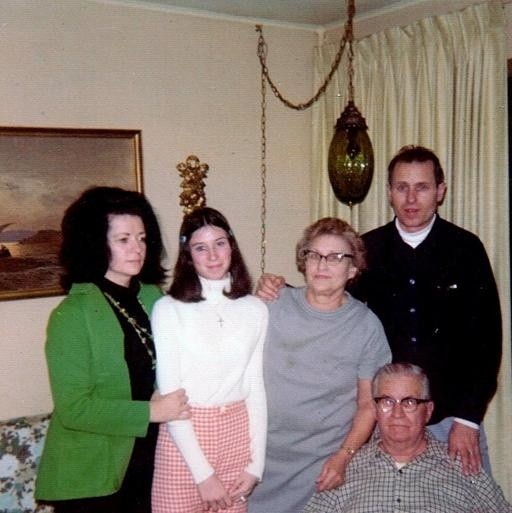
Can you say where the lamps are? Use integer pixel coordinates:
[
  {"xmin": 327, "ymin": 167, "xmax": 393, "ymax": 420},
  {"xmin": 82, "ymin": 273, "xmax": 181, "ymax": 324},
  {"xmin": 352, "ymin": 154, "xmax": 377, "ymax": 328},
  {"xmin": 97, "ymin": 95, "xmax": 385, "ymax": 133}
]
[{"xmin": 328, "ymin": 100, "xmax": 374, "ymax": 207}]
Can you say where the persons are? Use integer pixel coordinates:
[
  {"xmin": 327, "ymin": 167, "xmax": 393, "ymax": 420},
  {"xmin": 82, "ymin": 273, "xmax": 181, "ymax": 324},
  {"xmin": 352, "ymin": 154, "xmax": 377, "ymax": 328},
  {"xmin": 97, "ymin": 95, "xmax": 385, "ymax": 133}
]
[
  {"xmin": 247, "ymin": 215, "xmax": 394, "ymax": 513},
  {"xmin": 303, "ymin": 361, "xmax": 512, "ymax": 513},
  {"xmin": 148, "ymin": 205, "xmax": 270, "ymax": 513},
  {"xmin": 253, "ymin": 145, "xmax": 503, "ymax": 478},
  {"xmin": 33, "ymin": 185, "xmax": 193, "ymax": 513}
]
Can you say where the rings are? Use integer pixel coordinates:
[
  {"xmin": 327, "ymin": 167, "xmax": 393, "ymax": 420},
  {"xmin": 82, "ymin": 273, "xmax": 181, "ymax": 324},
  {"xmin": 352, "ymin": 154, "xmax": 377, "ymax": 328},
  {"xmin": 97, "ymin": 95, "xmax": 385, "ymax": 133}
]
[{"xmin": 241, "ymin": 496, "xmax": 246, "ymax": 502}]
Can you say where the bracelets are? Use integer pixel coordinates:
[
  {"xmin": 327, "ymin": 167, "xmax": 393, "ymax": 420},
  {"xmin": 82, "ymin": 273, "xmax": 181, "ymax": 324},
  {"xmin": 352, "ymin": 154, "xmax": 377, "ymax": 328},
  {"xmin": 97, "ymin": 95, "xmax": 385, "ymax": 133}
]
[{"xmin": 341, "ymin": 444, "xmax": 356, "ymax": 458}]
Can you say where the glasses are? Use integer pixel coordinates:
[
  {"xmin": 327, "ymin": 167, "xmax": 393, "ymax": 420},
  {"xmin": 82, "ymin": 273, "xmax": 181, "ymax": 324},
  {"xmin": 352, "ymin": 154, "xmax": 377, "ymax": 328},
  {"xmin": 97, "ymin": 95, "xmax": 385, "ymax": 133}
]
[
  {"xmin": 372, "ymin": 396, "xmax": 430, "ymax": 413},
  {"xmin": 304, "ymin": 249, "xmax": 354, "ymax": 264}
]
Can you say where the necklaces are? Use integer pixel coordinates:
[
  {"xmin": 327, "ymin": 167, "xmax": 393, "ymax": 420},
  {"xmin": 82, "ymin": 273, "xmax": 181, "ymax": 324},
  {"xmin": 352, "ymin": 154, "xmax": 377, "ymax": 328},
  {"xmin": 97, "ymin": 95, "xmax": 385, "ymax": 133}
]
[
  {"xmin": 103, "ymin": 291, "xmax": 157, "ymax": 373},
  {"xmin": 204, "ymin": 297, "xmax": 230, "ymax": 327}
]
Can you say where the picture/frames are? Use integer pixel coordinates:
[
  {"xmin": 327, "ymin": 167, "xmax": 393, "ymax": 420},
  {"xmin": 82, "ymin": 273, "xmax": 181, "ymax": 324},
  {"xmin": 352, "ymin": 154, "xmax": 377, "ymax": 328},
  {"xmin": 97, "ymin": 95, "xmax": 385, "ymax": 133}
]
[{"xmin": 0, "ymin": 126, "xmax": 144, "ymax": 300}]
